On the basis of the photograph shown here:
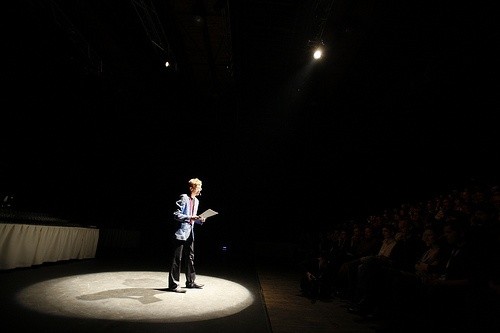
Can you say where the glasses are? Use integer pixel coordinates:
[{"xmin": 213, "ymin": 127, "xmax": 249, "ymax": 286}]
[{"xmin": 193, "ymin": 187, "xmax": 202, "ymax": 191}]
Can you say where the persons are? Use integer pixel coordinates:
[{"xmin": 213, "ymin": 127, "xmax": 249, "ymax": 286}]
[
  {"xmin": 333, "ymin": 179, "xmax": 499, "ymax": 293},
  {"xmin": 169, "ymin": 178, "xmax": 200, "ymax": 292}
]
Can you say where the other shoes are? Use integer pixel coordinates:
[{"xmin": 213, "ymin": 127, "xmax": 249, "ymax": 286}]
[
  {"xmin": 186, "ymin": 283, "xmax": 203, "ymax": 288},
  {"xmin": 170, "ymin": 287, "xmax": 186, "ymax": 293}
]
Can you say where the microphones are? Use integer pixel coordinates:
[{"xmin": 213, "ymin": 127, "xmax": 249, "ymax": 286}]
[{"xmin": 199, "ymin": 193, "xmax": 201, "ymax": 196}]
